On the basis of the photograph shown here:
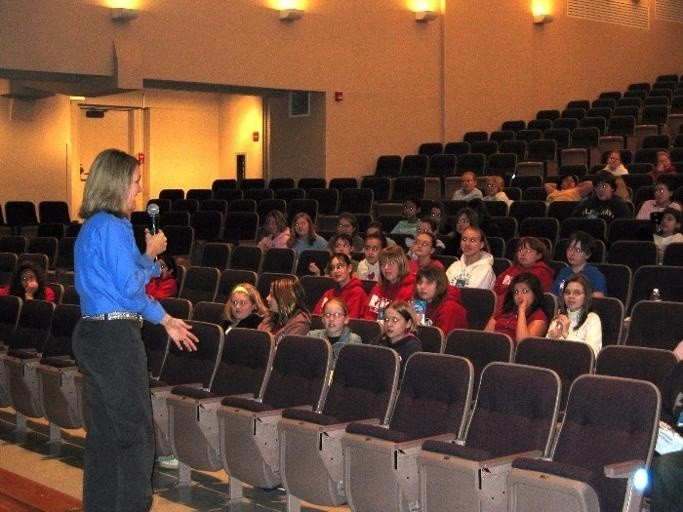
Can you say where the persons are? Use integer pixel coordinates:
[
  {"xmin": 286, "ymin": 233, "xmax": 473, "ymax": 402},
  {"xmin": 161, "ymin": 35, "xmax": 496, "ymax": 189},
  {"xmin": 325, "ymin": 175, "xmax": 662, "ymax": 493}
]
[
  {"xmin": 450, "ymin": 170, "xmax": 514, "ymax": 207},
  {"xmin": 1, "ymin": 259, "xmax": 56, "ymax": 304},
  {"xmin": 542, "ymin": 153, "xmax": 683, "ymax": 265},
  {"xmin": 145, "ymin": 253, "xmax": 180, "ymax": 299},
  {"xmin": 645, "ymin": 325, "xmax": 683, "ymax": 456},
  {"xmin": 69, "ymin": 148, "xmax": 198, "ymax": 511},
  {"xmin": 220, "ymin": 200, "xmax": 609, "ymax": 363}
]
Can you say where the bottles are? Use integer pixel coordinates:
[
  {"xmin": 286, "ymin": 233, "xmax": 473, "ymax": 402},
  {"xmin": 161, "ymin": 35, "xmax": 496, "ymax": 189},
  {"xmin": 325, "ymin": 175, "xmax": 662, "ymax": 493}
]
[{"xmin": 649, "ymin": 288, "xmax": 661, "ymax": 302}]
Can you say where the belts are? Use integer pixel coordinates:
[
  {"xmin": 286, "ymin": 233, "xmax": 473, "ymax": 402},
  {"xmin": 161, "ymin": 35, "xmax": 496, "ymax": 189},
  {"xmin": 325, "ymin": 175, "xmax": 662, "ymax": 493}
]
[{"xmin": 78, "ymin": 311, "xmax": 144, "ymax": 322}]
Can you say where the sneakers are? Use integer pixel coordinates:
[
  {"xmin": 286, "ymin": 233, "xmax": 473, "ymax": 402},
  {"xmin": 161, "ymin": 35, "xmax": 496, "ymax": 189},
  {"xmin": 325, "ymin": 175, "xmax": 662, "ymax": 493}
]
[{"xmin": 158, "ymin": 454, "xmax": 179, "ymax": 469}]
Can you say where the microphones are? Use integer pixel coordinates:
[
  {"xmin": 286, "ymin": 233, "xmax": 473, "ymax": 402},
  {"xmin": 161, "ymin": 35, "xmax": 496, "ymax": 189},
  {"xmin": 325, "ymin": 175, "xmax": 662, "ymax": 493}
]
[{"xmin": 146, "ymin": 203, "xmax": 160, "ymax": 261}]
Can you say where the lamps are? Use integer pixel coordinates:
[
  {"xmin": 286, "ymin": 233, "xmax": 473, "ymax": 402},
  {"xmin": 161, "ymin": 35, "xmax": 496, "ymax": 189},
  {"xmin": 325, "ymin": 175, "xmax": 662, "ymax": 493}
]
[
  {"xmin": 531, "ymin": 1, "xmax": 554, "ymax": 25},
  {"xmin": 406, "ymin": 1, "xmax": 441, "ymax": 21},
  {"xmin": 266, "ymin": 0, "xmax": 308, "ymax": 22},
  {"xmin": 105, "ymin": 2, "xmax": 139, "ymax": 22}
]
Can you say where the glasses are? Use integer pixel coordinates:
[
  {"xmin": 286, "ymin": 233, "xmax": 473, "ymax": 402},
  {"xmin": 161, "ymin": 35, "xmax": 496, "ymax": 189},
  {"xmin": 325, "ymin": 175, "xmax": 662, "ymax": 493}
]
[{"xmin": 324, "ymin": 312, "xmax": 343, "ymax": 318}]
